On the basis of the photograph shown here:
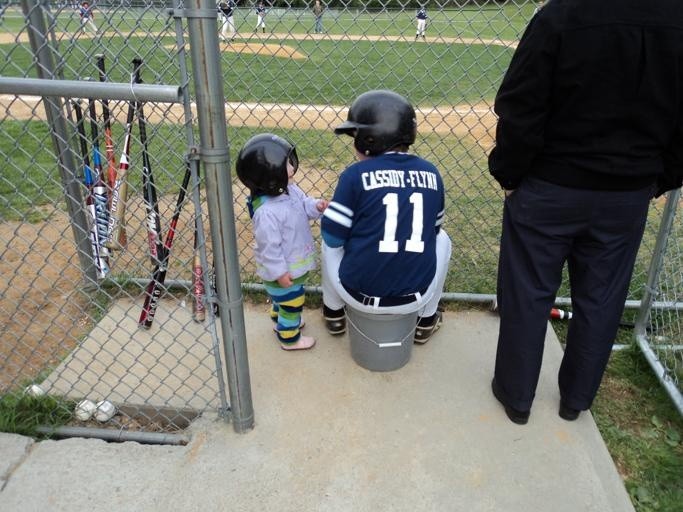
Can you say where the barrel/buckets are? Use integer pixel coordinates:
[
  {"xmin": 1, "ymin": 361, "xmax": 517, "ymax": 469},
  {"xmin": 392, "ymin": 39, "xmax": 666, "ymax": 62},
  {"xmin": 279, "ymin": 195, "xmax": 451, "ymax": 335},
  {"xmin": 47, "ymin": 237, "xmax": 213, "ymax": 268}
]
[{"xmin": 344, "ymin": 302, "xmax": 419, "ymax": 372}]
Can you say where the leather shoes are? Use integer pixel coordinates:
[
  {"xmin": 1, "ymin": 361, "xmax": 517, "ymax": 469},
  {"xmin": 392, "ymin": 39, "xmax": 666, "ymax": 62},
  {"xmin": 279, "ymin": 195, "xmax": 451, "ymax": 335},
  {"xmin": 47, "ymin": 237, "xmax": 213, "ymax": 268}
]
[
  {"xmin": 559, "ymin": 400, "xmax": 580, "ymax": 422},
  {"xmin": 492, "ymin": 377, "xmax": 530, "ymax": 425}
]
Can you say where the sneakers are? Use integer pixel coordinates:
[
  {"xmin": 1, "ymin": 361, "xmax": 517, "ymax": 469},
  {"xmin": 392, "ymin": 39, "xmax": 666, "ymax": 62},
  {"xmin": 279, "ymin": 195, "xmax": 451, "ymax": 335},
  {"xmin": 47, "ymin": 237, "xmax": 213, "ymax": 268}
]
[
  {"xmin": 414, "ymin": 311, "xmax": 442, "ymax": 344},
  {"xmin": 323, "ymin": 311, "xmax": 347, "ymax": 335},
  {"xmin": 273, "ymin": 319, "xmax": 317, "ymax": 350}
]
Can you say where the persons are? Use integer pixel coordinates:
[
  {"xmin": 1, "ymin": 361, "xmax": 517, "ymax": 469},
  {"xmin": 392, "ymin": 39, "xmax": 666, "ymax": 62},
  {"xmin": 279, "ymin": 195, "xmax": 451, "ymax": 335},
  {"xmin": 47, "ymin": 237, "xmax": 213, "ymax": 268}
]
[
  {"xmin": 484, "ymin": 0, "xmax": 683, "ymax": 424},
  {"xmin": 413, "ymin": 6, "xmax": 427, "ymax": 39},
  {"xmin": 234, "ymin": 132, "xmax": 331, "ymax": 351},
  {"xmin": 320, "ymin": 88, "xmax": 453, "ymax": 345},
  {"xmin": 254, "ymin": 2, "xmax": 267, "ymax": 33},
  {"xmin": 78, "ymin": 2, "xmax": 97, "ymax": 35},
  {"xmin": 311, "ymin": 1, "xmax": 323, "ymax": 35},
  {"xmin": 217, "ymin": 0, "xmax": 235, "ymax": 42}
]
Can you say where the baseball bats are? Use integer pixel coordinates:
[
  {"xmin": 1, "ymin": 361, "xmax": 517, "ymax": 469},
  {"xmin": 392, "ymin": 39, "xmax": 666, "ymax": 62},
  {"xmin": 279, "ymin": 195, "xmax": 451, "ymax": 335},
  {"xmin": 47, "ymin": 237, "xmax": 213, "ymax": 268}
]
[
  {"xmin": 490, "ymin": 300, "xmax": 661, "ymax": 331},
  {"xmin": 71, "ymin": 54, "xmax": 219, "ymax": 330}
]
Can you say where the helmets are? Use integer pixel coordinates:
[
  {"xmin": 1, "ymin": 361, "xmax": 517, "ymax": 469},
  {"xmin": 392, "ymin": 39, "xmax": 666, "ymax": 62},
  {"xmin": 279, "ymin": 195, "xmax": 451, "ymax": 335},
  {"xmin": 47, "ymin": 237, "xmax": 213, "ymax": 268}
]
[
  {"xmin": 335, "ymin": 90, "xmax": 417, "ymax": 158},
  {"xmin": 236, "ymin": 132, "xmax": 300, "ymax": 197}
]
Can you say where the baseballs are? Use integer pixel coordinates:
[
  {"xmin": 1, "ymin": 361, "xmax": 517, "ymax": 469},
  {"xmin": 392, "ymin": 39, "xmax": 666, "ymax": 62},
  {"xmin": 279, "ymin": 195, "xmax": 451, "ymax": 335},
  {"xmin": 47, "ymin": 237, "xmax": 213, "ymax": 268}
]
[
  {"xmin": 21, "ymin": 384, "xmax": 42, "ymax": 397},
  {"xmin": 72, "ymin": 400, "xmax": 116, "ymax": 423}
]
